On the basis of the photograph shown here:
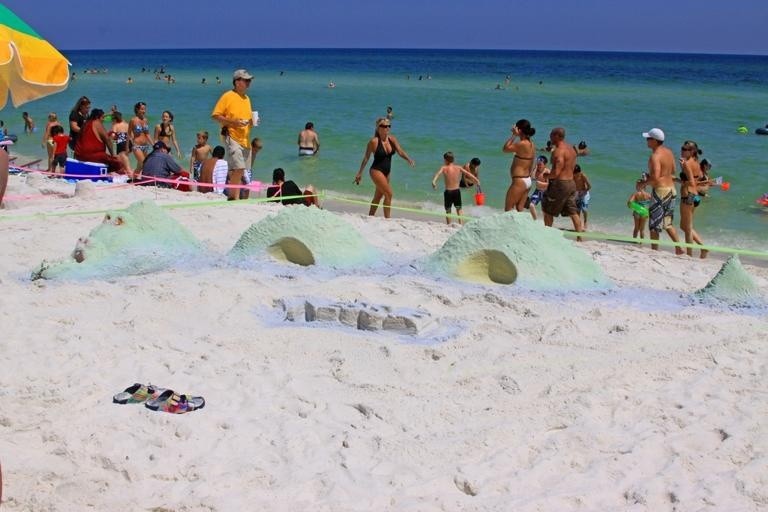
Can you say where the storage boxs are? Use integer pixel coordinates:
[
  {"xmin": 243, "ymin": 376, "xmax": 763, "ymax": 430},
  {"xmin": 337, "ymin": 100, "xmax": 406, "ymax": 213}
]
[{"xmin": 62, "ymin": 157, "xmax": 114, "ymax": 185}]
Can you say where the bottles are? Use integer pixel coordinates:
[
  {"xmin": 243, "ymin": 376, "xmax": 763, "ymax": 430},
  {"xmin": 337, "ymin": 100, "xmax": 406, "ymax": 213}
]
[{"xmin": 112, "ymin": 140, "xmax": 117, "ymax": 154}]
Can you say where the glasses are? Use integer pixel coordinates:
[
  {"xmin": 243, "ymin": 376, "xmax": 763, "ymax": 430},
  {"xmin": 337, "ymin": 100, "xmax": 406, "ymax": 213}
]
[
  {"xmin": 379, "ymin": 124, "xmax": 391, "ymax": 128},
  {"xmin": 237, "ymin": 78, "xmax": 251, "ymax": 82},
  {"xmin": 681, "ymin": 146, "xmax": 692, "ymax": 151}
]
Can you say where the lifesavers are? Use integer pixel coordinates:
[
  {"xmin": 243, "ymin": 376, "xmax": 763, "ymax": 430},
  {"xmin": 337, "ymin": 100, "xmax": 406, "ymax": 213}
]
[
  {"xmin": 735, "ymin": 126, "xmax": 747, "ymax": 135},
  {"xmin": 629, "ymin": 200, "xmax": 649, "ymax": 219},
  {"xmin": 754, "ymin": 193, "xmax": 768, "ymax": 206}
]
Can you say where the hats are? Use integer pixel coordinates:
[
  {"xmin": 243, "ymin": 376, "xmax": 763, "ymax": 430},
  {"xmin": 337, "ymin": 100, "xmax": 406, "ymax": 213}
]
[
  {"xmin": 232, "ymin": 68, "xmax": 254, "ymax": 80},
  {"xmin": 641, "ymin": 127, "xmax": 665, "ymax": 141},
  {"xmin": 153, "ymin": 141, "xmax": 171, "ymax": 153}
]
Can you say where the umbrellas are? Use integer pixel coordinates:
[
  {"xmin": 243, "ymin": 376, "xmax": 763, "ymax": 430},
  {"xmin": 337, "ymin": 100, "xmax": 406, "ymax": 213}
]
[{"xmin": 0, "ymin": 2, "xmax": 72, "ymax": 110}]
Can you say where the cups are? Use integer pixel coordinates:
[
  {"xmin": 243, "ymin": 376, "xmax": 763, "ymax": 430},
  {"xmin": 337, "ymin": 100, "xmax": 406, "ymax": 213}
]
[{"xmin": 251, "ymin": 111, "xmax": 259, "ymax": 126}]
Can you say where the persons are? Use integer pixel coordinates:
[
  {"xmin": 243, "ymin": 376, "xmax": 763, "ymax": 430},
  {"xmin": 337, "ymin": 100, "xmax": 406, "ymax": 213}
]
[
  {"xmin": 458, "ymin": 158, "xmax": 480, "ymax": 187},
  {"xmin": 72, "ymin": 63, "xmax": 175, "ymax": 84},
  {"xmin": 200, "ymin": 78, "xmax": 208, "ymax": 85},
  {"xmin": 699, "ymin": 158, "xmax": 716, "ymax": 186},
  {"xmin": 639, "ymin": 128, "xmax": 684, "ymax": 255},
  {"xmin": 328, "ymin": 80, "xmax": 336, "ymax": 88},
  {"xmin": 351, "ymin": 118, "xmax": 414, "ymax": 217},
  {"xmin": 0, "ymin": 120, "xmax": 7, "ymax": 152},
  {"xmin": 210, "ymin": 69, "xmax": 259, "ymax": 201},
  {"xmin": 22, "ymin": 112, "xmax": 34, "ymax": 134},
  {"xmin": 502, "ymin": 118, "xmax": 592, "ymax": 232},
  {"xmin": 266, "ymin": 167, "xmax": 318, "ymax": 207},
  {"xmin": 41, "ymin": 96, "xmax": 262, "ymax": 199},
  {"xmin": 673, "ymin": 141, "xmax": 709, "ymax": 258},
  {"xmin": 627, "ymin": 179, "xmax": 651, "ymax": 239},
  {"xmin": 0, "ymin": 147, "xmax": 8, "ymax": 207},
  {"xmin": 505, "ymin": 74, "xmax": 511, "ymax": 88},
  {"xmin": 297, "ymin": 122, "xmax": 320, "ymax": 156},
  {"xmin": 431, "ymin": 152, "xmax": 479, "ymax": 225},
  {"xmin": 215, "ymin": 76, "xmax": 221, "ymax": 85}
]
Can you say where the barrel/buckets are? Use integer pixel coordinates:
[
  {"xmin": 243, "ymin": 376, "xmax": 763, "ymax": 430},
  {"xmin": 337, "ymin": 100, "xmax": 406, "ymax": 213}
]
[{"xmin": 475, "ymin": 184, "xmax": 485, "ymax": 206}]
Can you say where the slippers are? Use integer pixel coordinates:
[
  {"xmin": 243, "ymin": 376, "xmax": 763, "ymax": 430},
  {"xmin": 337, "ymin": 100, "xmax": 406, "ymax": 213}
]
[
  {"xmin": 111, "ymin": 383, "xmax": 168, "ymax": 406},
  {"xmin": 145, "ymin": 389, "xmax": 206, "ymax": 413}
]
